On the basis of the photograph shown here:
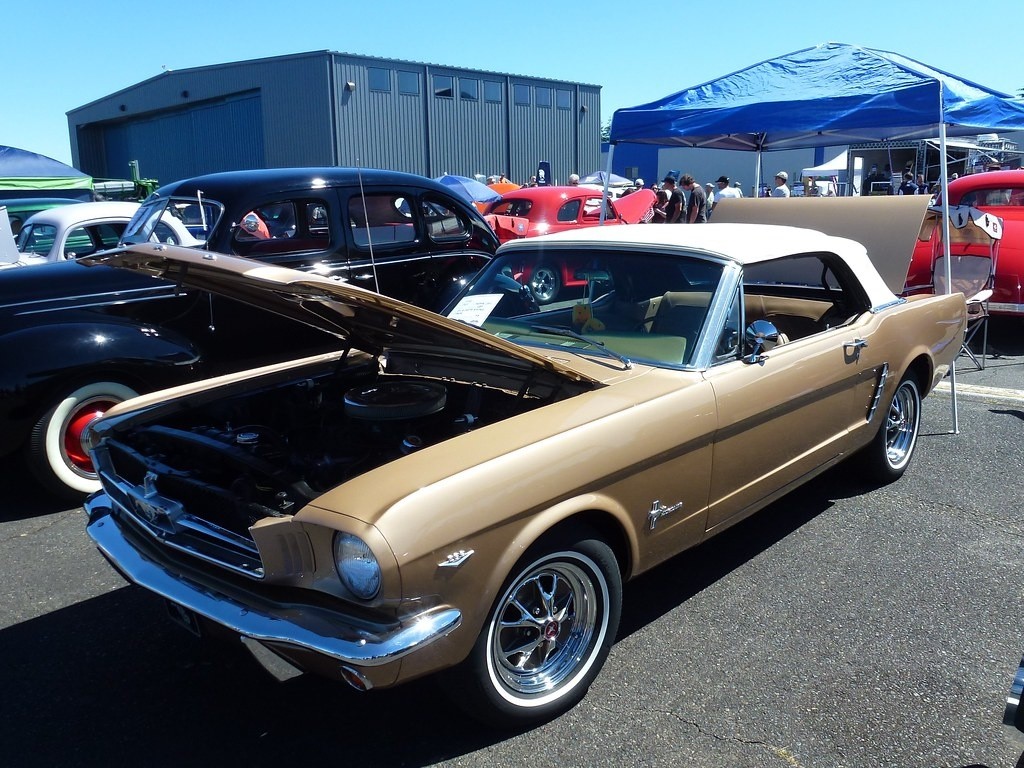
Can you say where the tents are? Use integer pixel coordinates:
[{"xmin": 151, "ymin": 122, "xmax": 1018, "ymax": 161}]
[
  {"xmin": 577, "ymin": 170, "xmax": 634, "ymax": 188},
  {"xmin": 597, "ymin": 41, "xmax": 1024, "ymax": 434},
  {"xmin": 0, "ymin": 144, "xmax": 95, "ymax": 204},
  {"xmin": 801, "ymin": 147, "xmax": 864, "ymax": 197}
]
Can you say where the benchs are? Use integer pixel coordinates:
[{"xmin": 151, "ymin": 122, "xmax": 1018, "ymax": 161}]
[
  {"xmin": 656, "ymin": 291, "xmax": 840, "ymax": 348},
  {"xmin": 252, "ymin": 239, "xmax": 327, "ymax": 254}
]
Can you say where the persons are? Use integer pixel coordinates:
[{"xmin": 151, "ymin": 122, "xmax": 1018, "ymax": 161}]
[
  {"xmin": 486, "ymin": 172, "xmax": 580, "ymax": 190},
  {"xmin": 635, "ymin": 170, "xmax": 790, "ymax": 224},
  {"xmin": 862, "ymin": 161, "xmax": 959, "ymax": 195}
]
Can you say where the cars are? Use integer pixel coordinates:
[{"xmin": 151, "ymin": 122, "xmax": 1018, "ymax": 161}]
[
  {"xmin": 899, "ymin": 170, "xmax": 1024, "ymax": 317},
  {"xmin": 0, "ymin": 167, "xmax": 500, "ymax": 506},
  {"xmin": 433, "ymin": 185, "xmax": 657, "ymax": 252},
  {"xmin": 76, "ymin": 159, "xmax": 967, "ymax": 723}
]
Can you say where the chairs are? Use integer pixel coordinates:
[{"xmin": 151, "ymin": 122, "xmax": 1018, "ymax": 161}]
[{"xmin": 903, "ymin": 204, "xmax": 1005, "ymax": 371}]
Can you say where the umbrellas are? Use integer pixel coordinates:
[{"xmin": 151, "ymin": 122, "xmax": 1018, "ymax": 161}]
[{"xmin": 398, "ymin": 169, "xmax": 504, "ymax": 215}]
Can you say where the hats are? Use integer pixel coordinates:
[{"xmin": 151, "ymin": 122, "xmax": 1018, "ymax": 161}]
[
  {"xmin": 661, "ymin": 175, "xmax": 675, "ymax": 183},
  {"xmin": 635, "ymin": 178, "xmax": 644, "ymax": 187},
  {"xmin": 705, "ymin": 183, "xmax": 716, "ymax": 188},
  {"xmin": 905, "ymin": 160, "xmax": 915, "ymax": 167},
  {"xmin": 986, "ymin": 158, "xmax": 1000, "ymax": 167},
  {"xmin": 773, "ymin": 172, "xmax": 788, "ymax": 180},
  {"xmin": 715, "ymin": 175, "xmax": 730, "ymax": 183},
  {"xmin": 734, "ymin": 182, "xmax": 741, "ymax": 185},
  {"xmin": 951, "ymin": 173, "xmax": 958, "ymax": 178}
]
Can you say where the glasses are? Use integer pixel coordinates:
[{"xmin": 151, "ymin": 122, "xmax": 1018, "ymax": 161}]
[{"xmin": 572, "ymin": 183, "xmax": 578, "ymax": 184}]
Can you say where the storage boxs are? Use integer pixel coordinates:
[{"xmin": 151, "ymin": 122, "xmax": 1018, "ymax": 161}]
[
  {"xmin": 752, "ymin": 182, "xmax": 767, "ymax": 197},
  {"xmin": 794, "ymin": 182, "xmax": 804, "ymax": 191},
  {"xmin": 791, "ymin": 190, "xmax": 805, "ymax": 197}
]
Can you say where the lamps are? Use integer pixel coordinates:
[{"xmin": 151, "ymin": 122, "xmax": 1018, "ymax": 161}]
[
  {"xmin": 347, "ymin": 83, "xmax": 354, "ymax": 92},
  {"xmin": 119, "ymin": 104, "xmax": 126, "ymax": 111},
  {"xmin": 581, "ymin": 105, "xmax": 588, "ymax": 113}
]
